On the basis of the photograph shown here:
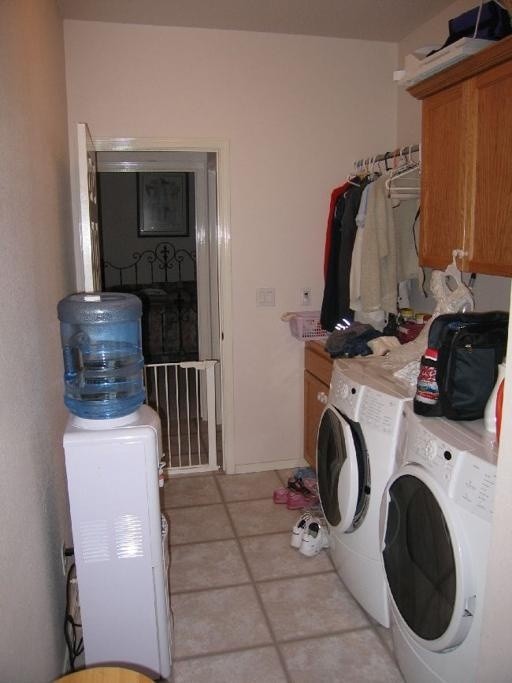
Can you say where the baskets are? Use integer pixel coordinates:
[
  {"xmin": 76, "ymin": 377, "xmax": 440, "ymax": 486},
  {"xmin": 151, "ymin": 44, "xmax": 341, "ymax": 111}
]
[{"xmin": 289, "ymin": 311, "xmax": 332, "ymax": 342}]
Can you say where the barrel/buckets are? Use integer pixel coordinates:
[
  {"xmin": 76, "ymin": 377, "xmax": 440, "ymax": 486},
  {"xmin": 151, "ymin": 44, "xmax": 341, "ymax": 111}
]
[{"xmin": 55, "ymin": 290, "xmax": 147, "ymax": 420}]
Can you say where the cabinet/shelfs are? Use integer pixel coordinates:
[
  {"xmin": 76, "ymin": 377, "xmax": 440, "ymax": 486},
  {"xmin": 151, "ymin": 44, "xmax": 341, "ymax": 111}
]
[
  {"xmin": 407, "ymin": 58, "xmax": 512, "ymax": 277},
  {"xmin": 299, "ymin": 337, "xmax": 341, "ymax": 475}
]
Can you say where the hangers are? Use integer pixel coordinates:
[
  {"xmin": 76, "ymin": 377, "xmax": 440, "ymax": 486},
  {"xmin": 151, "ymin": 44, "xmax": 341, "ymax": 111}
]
[{"xmin": 342, "ymin": 139, "xmax": 426, "ymax": 198}]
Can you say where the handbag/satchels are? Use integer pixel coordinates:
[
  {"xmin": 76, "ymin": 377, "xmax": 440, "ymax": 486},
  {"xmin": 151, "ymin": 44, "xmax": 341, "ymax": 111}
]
[{"xmin": 414, "ymin": 310, "xmax": 509, "ymax": 421}]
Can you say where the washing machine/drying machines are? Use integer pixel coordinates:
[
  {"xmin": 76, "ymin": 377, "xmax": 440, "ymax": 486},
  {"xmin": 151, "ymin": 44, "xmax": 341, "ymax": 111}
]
[
  {"xmin": 378, "ymin": 394, "xmax": 498, "ymax": 683},
  {"xmin": 315, "ymin": 357, "xmax": 416, "ymax": 629}
]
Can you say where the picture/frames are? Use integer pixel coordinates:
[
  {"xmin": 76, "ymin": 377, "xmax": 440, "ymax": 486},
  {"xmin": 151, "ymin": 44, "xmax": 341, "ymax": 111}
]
[{"xmin": 136, "ymin": 172, "xmax": 189, "ymax": 237}]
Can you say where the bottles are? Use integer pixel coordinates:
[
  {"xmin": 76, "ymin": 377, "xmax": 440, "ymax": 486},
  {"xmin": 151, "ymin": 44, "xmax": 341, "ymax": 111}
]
[{"xmin": 415, "ymin": 348, "xmax": 440, "ymax": 405}]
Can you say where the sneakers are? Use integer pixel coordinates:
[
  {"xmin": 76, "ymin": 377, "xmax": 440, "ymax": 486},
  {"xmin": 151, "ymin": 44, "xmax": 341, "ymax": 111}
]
[
  {"xmin": 273, "ymin": 486, "xmax": 318, "ymax": 510},
  {"xmin": 287, "ymin": 476, "xmax": 311, "ymax": 496},
  {"xmin": 291, "ymin": 510, "xmax": 329, "ymax": 556}
]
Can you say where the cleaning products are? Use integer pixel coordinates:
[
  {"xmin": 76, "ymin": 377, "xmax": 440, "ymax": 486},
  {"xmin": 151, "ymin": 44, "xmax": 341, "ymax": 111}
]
[{"xmin": 484, "ymin": 356, "xmax": 506, "ymax": 433}]
